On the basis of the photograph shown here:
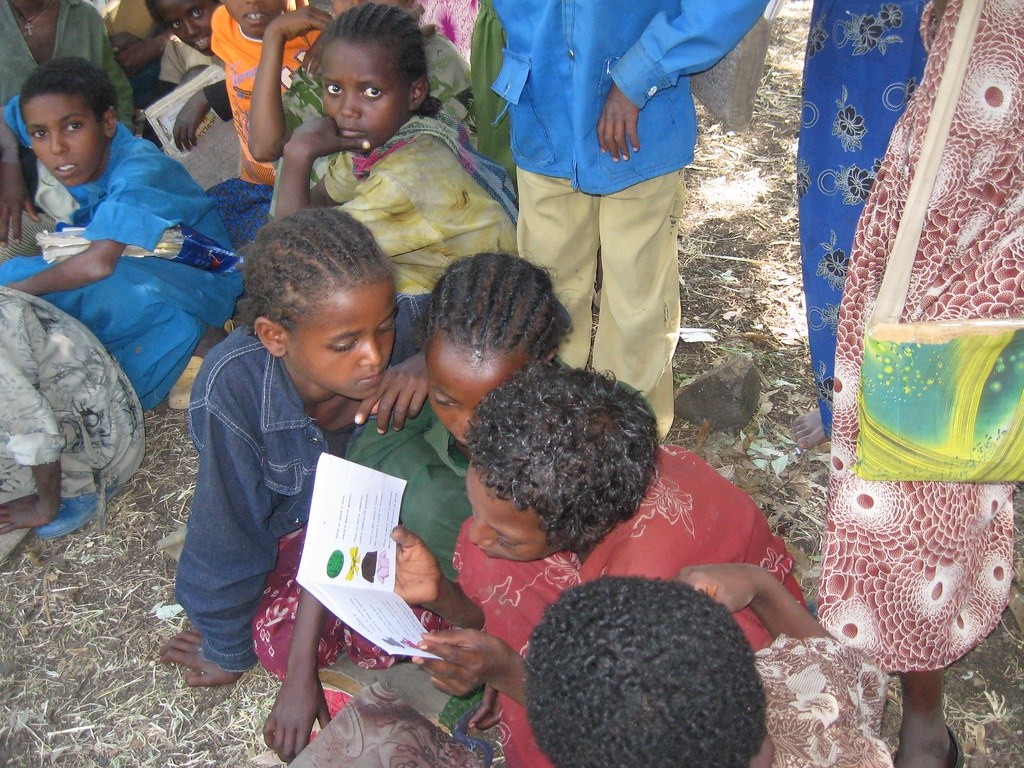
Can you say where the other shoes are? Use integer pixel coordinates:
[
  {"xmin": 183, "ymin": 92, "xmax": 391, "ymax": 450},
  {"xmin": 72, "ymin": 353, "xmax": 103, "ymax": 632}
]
[
  {"xmin": 893, "ymin": 724, "xmax": 964, "ymax": 768},
  {"xmin": 34, "ymin": 485, "xmax": 119, "ymax": 538},
  {"xmin": 169, "ymin": 319, "xmax": 234, "ymax": 410}
]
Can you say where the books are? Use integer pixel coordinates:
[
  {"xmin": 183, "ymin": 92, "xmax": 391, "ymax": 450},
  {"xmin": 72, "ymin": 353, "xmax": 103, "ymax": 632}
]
[{"xmin": 296, "ymin": 450, "xmax": 444, "ymax": 660}]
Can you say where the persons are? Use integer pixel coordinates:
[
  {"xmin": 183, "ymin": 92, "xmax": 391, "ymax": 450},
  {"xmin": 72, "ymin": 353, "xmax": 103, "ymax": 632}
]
[
  {"xmin": 0, "ymin": 55, "xmax": 243, "ymax": 410},
  {"xmin": 491, "ymin": 0, "xmax": 772, "ymax": 447},
  {"xmin": 246, "ymin": 0, "xmax": 480, "ymax": 225},
  {"xmin": 521, "ymin": 563, "xmax": 896, "ymax": 768},
  {"xmin": 95, "ymin": 0, "xmax": 172, "ymax": 113},
  {"xmin": 0, "ymin": 284, "xmax": 146, "ymax": 541},
  {"xmin": 273, "ymin": 2, "xmax": 518, "ymax": 296},
  {"xmin": 390, "ymin": 357, "xmax": 807, "ymax": 768},
  {"xmin": 143, "ymin": 0, "xmax": 233, "ymax": 151},
  {"xmin": 159, "ymin": 206, "xmax": 432, "ymax": 687},
  {"xmin": 0, "ymin": 0, "xmax": 132, "ymax": 248},
  {"xmin": 261, "ymin": 250, "xmax": 576, "ymax": 767},
  {"xmin": 205, "ymin": 0, "xmax": 321, "ymax": 251},
  {"xmin": 796, "ymin": 0, "xmax": 1024, "ymax": 768}
]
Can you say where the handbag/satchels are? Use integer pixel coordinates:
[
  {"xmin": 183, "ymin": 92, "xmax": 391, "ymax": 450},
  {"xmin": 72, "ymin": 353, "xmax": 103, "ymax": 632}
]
[{"xmin": 855, "ymin": 319, "xmax": 1024, "ymax": 481}]
